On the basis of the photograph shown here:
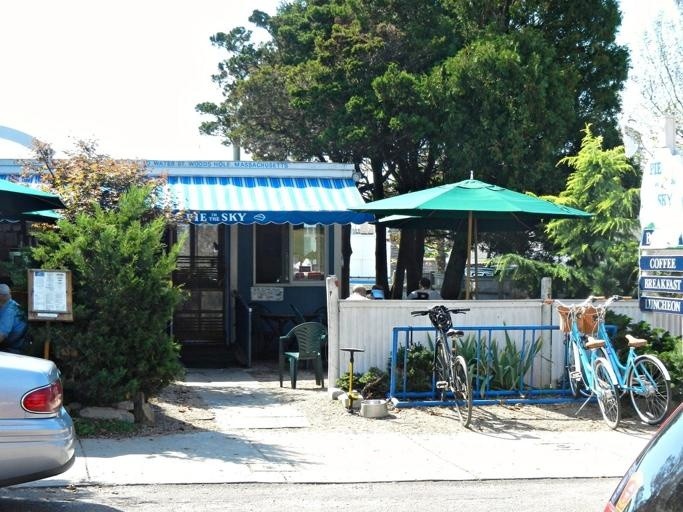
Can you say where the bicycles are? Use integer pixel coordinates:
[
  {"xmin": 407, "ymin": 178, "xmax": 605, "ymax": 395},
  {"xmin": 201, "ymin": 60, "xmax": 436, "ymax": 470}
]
[
  {"xmin": 544, "ymin": 295, "xmax": 672, "ymax": 430},
  {"xmin": 411, "ymin": 308, "xmax": 473, "ymax": 428}
]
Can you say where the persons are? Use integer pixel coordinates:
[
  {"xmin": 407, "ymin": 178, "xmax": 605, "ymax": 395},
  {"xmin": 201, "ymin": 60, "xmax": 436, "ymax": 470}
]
[
  {"xmin": 406, "ymin": 278, "xmax": 443, "ymax": 298},
  {"xmin": 0, "ymin": 283, "xmax": 26, "ymax": 352},
  {"xmin": 371, "ymin": 285, "xmax": 388, "ymax": 299},
  {"xmin": 345, "ymin": 286, "xmax": 367, "ymax": 299}
]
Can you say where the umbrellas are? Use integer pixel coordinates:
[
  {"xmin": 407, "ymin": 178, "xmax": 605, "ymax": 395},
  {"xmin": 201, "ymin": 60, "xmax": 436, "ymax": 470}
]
[
  {"xmin": 370, "ymin": 213, "xmax": 553, "ymax": 299},
  {"xmin": 346, "ymin": 174, "xmax": 590, "ymax": 299},
  {"xmin": 0, "ymin": 177, "xmax": 71, "ymax": 223},
  {"xmin": 13, "ymin": 203, "xmax": 59, "ymax": 223}
]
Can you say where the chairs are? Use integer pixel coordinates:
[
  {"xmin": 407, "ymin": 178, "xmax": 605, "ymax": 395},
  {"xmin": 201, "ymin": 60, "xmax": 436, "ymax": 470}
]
[{"xmin": 251, "ymin": 302, "xmax": 328, "ymax": 389}]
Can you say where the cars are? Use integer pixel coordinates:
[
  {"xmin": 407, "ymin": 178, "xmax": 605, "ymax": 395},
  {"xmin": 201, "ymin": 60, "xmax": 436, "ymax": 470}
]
[
  {"xmin": 604, "ymin": 402, "xmax": 683, "ymax": 512},
  {"xmin": 0, "ymin": 352, "xmax": 77, "ymax": 487}
]
